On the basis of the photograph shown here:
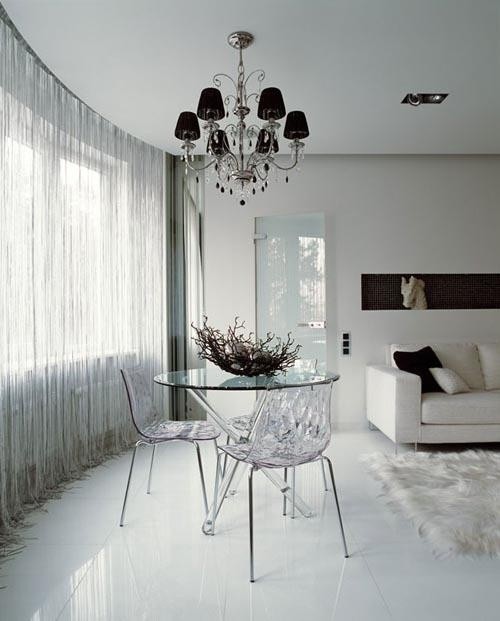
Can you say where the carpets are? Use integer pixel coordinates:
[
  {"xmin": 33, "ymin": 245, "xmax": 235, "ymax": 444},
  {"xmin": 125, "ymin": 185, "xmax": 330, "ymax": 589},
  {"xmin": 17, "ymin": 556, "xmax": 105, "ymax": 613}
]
[{"xmin": 361, "ymin": 449, "xmax": 500, "ymax": 563}]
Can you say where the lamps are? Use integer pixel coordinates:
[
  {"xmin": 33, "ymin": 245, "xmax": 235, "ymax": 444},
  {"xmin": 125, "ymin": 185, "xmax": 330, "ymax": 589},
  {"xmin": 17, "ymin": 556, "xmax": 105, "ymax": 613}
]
[{"xmin": 174, "ymin": 32, "xmax": 310, "ymax": 207}]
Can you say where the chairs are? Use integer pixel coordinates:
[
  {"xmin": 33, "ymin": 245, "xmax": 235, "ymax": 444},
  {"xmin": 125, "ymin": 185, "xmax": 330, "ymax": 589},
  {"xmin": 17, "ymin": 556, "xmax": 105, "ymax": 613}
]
[
  {"xmin": 217, "ymin": 357, "xmax": 328, "ymax": 518},
  {"xmin": 115, "ymin": 367, "xmax": 224, "ymax": 527},
  {"xmin": 204, "ymin": 376, "xmax": 354, "ymax": 583}
]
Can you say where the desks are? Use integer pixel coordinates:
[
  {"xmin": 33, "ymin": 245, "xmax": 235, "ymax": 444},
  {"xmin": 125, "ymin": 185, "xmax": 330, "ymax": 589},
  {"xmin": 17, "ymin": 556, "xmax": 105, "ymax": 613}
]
[{"xmin": 155, "ymin": 365, "xmax": 341, "ymax": 520}]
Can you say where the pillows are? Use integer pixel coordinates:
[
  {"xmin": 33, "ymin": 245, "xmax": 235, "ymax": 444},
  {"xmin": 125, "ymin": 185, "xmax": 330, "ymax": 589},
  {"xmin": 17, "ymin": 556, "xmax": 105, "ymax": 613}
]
[
  {"xmin": 476, "ymin": 343, "xmax": 500, "ymax": 393},
  {"xmin": 431, "ymin": 367, "xmax": 472, "ymax": 397},
  {"xmin": 392, "ymin": 346, "xmax": 447, "ymax": 392}
]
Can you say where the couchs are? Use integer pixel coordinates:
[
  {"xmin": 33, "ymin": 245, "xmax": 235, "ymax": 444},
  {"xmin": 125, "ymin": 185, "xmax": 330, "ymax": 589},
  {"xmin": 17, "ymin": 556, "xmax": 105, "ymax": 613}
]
[{"xmin": 366, "ymin": 341, "xmax": 500, "ymax": 455}]
